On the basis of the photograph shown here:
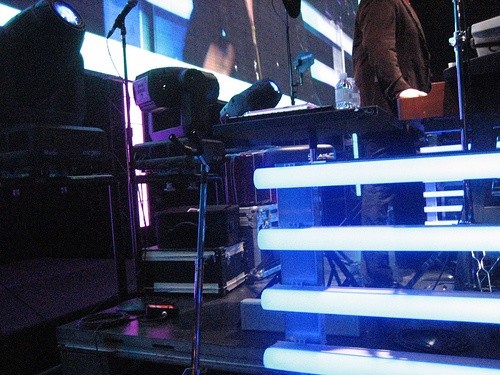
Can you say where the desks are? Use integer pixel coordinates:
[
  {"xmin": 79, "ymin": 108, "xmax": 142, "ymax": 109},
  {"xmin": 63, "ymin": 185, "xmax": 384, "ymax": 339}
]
[{"xmin": 0, "ymin": 172, "xmax": 119, "ymax": 337}]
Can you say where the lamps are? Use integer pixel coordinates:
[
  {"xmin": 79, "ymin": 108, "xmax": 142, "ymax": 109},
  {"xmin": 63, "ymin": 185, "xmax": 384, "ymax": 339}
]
[
  {"xmin": 221, "ymin": 79, "xmax": 281, "ymax": 122},
  {"xmin": 0, "ymin": 0, "xmax": 86, "ymax": 125}
]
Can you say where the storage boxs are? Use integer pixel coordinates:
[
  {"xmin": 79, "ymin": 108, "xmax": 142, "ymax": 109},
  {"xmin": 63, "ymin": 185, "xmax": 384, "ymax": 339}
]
[
  {"xmin": 224, "ymin": 150, "xmax": 276, "ymax": 202},
  {"xmin": 155, "ymin": 204, "xmax": 239, "ymax": 246},
  {"xmin": 239, "ymin": 203, "xmax": 280, "ymax": 278},
  {"xmin": 141, "ymin": 243, "xmax": 249, "ymax": 295}
]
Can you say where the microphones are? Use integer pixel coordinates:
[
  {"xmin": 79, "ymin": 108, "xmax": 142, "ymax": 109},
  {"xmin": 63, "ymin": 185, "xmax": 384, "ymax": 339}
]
[{"xmin": 106, "ymin": 1, "xmax": 137, "ymax": 40}]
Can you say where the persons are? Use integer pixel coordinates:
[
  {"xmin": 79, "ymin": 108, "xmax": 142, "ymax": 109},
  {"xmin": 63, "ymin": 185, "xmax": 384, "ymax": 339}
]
[{"xmin": 352, "ymin": 2, "xmax": 438, "ymax": 292}]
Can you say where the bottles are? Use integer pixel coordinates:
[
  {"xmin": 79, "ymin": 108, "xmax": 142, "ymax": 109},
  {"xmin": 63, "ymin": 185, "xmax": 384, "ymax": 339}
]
[
  {"xmin": 335, "ymin": 73, "xmax": 352, "ymax": 110},
  {"xmin": 348, "ymin": 80, "xmax": 361, "ymax": 108}
]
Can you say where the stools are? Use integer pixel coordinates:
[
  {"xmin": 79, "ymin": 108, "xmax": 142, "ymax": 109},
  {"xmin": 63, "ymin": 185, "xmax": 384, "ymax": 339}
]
[{"xmin": 249, "ymin": 144, "xmax": 360, "ymax": 296}]
